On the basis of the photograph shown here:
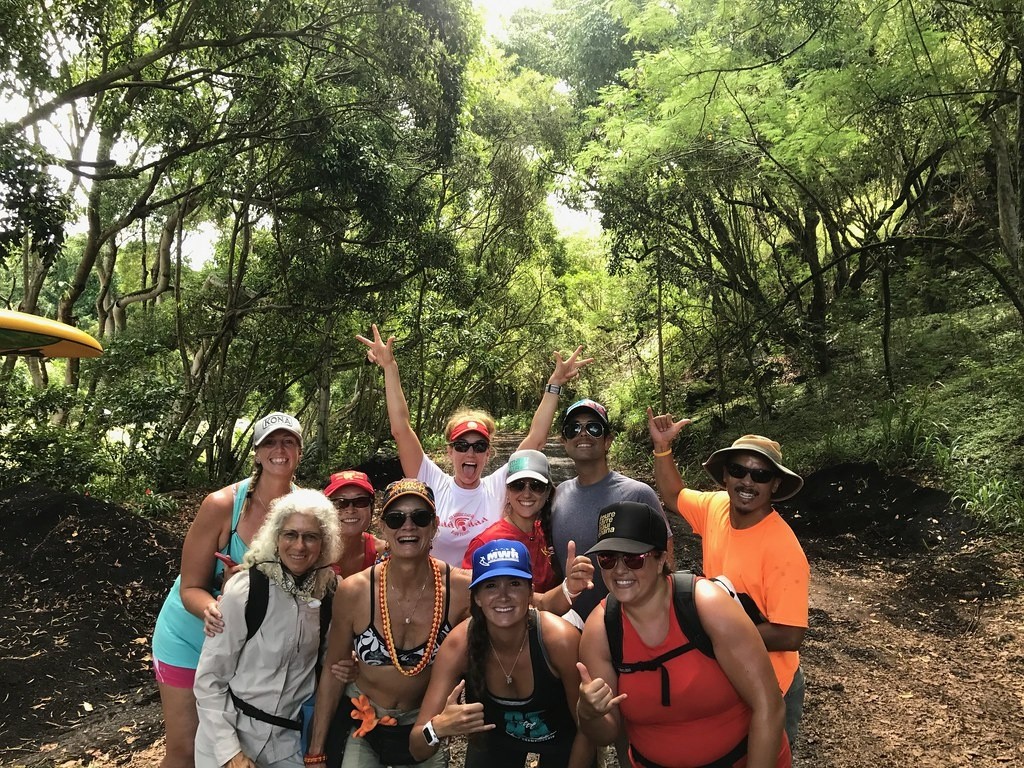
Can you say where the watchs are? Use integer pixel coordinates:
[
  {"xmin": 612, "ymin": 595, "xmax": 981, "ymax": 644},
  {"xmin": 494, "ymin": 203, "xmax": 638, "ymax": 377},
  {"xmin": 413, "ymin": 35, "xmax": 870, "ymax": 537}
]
[{"xmin": 422, "ymin": 714, "xmax": 440, "ymax": 746}]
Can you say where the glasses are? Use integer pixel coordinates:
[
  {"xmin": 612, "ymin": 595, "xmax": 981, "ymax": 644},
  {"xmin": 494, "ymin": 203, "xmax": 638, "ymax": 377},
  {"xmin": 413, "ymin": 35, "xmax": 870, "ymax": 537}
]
[
  {"xmin": 597, "ymin": 552, "xmax": 657, "ymax": 568},
  {"xmin": 382, "ymin": 511, "xmax": 436, "ymax": 529},
  {"xmin": 561, "ymin": 422, "xmax": 605, "ymax": 440},
  {"xmin": 277, "ymin": 528, "xmax": 323, "ymax": 544},
  {"xmin": 330, "ymin": 497, "xmax": 374, "ymax": 510},
  {"xmin": 509, "ymin": 480, "xmax": 547, "ymax": 494},
  {"xmin": 449, "ymin": 440, "xmax": 489, "ymax": 452},
  {"xmin": 723, "ymin": 461, "xmax": 777, "ymax": 483}
]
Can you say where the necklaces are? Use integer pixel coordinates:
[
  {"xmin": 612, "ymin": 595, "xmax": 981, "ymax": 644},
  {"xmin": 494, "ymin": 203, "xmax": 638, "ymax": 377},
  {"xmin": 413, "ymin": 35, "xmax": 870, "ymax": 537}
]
[
  {"xmin": 489, "ymin": 629, "xmax": 529, "ymax": 687},
  {"xmin": 389, "ymin": 576, "xmax": 429, "ymax": 624},
  {"xmin": 508, "ymin": 516, "xmax": 535, "ymax": 541}
]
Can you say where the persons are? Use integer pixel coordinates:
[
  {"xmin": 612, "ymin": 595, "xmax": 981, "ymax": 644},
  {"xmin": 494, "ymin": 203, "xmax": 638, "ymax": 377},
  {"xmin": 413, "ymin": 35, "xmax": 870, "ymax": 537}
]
[
  {"xmin": 303, "ymin": 477, "xmax": 595, "ymax": 768},
  {"xmin": 323, "ymin": 470, "xmax": 389, "ymax": 579},
  {"xmin": 461, "ymin": 449, "xmax": 565, "ymax": 595},
  {"xmin": 646, "ymin": 406, "xmax": 810, "ymax": 753},
  {"xmin": 193, "ymin": 488, "xmax": 360, "ymax": 768},
  {"xmin": 151, "ymin": 411, "xmax": 304, "ymax": 768},
  {"xmin": 355, "ymin": 323, "xmax": 594, "ymax": 568},
  {"xmin": 550, "ymin": 398, "xmax": 675, "ymax": 624},
  {"xmin": 575, "ymin": 500, "xmax": 792, "ymax": 768},
  {"xmin": 408, "ymin": 539, "xmax": 600, "ymax": 768}
]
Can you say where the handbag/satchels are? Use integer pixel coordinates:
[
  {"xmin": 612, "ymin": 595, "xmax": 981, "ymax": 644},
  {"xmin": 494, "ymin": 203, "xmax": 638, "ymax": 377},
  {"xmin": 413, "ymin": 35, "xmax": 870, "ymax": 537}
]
[{"xmin": 355, "ymin": 718, "xmax": 424, "ymax": 766}]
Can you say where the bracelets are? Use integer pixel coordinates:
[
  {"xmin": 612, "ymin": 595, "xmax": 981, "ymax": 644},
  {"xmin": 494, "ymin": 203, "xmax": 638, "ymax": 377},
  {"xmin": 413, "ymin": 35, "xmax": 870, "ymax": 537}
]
[
  {"xmin": 652, "ymin": 448, "xmax": 672, "ymax": 457},
  {"xmin": 304, "ymin": 754, "xmax": 328, "ymax": 765},
  {"xmin": 562, "ymin": 576, "xmax": 582, "ymax": 605},
  {"xmin": 545, "ymin": 383, "xmax": 564, "ymax": 396}
]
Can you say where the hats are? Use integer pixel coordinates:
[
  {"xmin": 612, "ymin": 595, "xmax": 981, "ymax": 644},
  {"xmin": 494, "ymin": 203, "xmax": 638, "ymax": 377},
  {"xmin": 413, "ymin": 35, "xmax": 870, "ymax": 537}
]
[
  {"xmin": 322, "ymin": 471, "xmax": 376, "ymax": 499},
  {"xmin": 252, "ymin": 411, "xmax": 303, "ymax": 442},
  {"xmin": 505, "ymin": 449, "xmax": 551, "ymax": 485},
  {"xmin": 380, "ymin": 477, "xmax": 436, "ymax": 510},
  {"xmin": 468, "ymin": 540, "xmax": 533, "ymax": 588},
  {"xmin": 562, "ymin": 399, "xmax": 609, "ymax": 425},
  {"xmin": 702, "ymin": 435, "xmax": 803, "ymax": 502},
  {"xmin": 580, "ymin": 500, "xmax": 669, "ymax": 555},
  {"xmin": 449, "ymin": 423, "xmax": 493, "ymax": 443}
]
